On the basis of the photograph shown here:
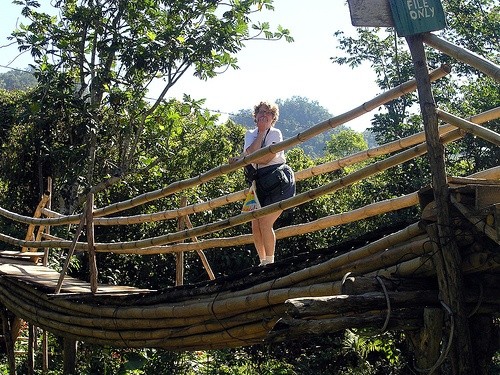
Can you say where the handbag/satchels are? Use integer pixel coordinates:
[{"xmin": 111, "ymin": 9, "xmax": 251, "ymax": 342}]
[{"xmin": 255, "ymin": 164, "xmax": 296, "ymax": 208}]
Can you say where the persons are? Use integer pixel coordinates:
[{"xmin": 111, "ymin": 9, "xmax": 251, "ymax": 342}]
[{"xmin": 227, "ymin": 102, "xmax": 295, "ymax": 267}]
